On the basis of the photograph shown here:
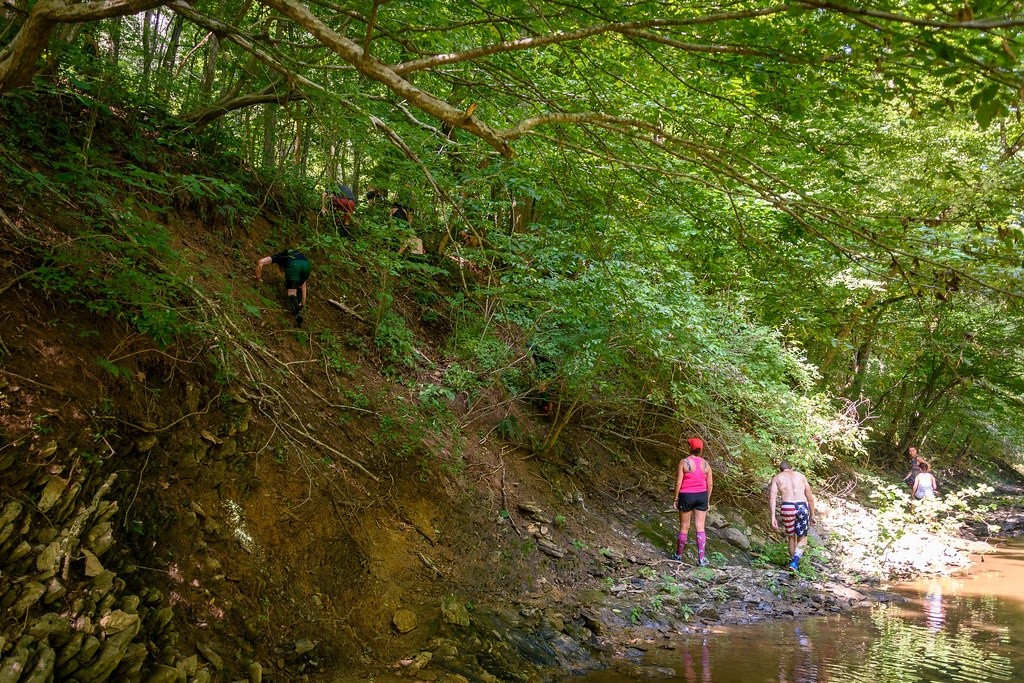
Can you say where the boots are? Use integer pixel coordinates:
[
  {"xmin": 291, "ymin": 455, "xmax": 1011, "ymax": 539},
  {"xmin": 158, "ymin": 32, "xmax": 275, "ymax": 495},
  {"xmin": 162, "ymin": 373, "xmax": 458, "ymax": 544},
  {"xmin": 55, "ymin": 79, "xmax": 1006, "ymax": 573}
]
[{"xmin": 288, "ymin": 288, "xmax": 303, "ymax": 315}]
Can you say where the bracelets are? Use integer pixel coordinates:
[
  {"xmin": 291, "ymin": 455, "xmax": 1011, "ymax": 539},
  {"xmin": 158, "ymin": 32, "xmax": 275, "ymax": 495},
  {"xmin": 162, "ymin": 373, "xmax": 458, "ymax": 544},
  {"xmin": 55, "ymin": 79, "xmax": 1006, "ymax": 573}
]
[{"xmin": 674, "ymin": 496, "xmax": 678, "ymax": 501}]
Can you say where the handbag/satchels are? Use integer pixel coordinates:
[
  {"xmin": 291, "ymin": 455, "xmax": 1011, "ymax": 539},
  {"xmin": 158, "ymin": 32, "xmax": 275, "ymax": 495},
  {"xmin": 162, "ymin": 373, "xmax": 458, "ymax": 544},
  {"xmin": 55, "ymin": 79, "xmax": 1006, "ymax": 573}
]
[{"xmin": 906, "ymin": 477, "xmax": 915, "ymax": 485}]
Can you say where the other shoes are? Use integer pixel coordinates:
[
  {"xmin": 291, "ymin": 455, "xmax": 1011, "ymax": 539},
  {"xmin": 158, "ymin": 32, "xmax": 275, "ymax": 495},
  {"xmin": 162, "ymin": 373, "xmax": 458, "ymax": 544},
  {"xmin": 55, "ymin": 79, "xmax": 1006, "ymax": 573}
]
[{"xmin": 344, "ymin": 225, "xmax": 349, "ymax": 236}]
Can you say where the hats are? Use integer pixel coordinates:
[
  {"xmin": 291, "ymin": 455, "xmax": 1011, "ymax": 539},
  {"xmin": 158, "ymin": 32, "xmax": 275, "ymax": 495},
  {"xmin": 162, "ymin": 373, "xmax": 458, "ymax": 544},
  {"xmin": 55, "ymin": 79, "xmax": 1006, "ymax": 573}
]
[
  {"xmin": 687, "ymin": 438, "xmax": 704, "ymax": 451},
  {"xmin": 780, "ymin": 461, "xmax": 791, "ymax": 470}
]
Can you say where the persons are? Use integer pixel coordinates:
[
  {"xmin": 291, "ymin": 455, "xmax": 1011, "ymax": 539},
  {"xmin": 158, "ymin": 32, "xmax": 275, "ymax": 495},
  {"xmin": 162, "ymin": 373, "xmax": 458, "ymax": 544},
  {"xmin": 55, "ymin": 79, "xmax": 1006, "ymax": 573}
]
[
  {"xmin": 902, "ymin": 446, "xmax": 937, "ymax": 501},
  {"xmin": 321, "ymin": 183, "xmax": 425, "ymax": 257},
  {"xmin": 674, "ymin": 437, "xmax": 714, "ymax": 566},
  {"xmin": 256, "ymin": 249, "xmax": 311, "ymax": 328},
  {"xmin": 770, "ymin": 461, "xmax": 817, "ymax": 573}
]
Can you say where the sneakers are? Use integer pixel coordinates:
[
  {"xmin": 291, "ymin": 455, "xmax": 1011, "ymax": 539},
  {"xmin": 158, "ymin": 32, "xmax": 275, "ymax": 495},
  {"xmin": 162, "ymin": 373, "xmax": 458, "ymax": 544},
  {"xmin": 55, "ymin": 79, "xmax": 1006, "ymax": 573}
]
[
  {"xmin": 789, "ymin": 562, "xmax": 799, "ymax": 571},
  {"xmin": 696, "ymin": 557, "xmax": 710, "ymax": 566},
  {"xmin": 671, "ymin": 554, "xmax": 682, "ymax": 562}
]
